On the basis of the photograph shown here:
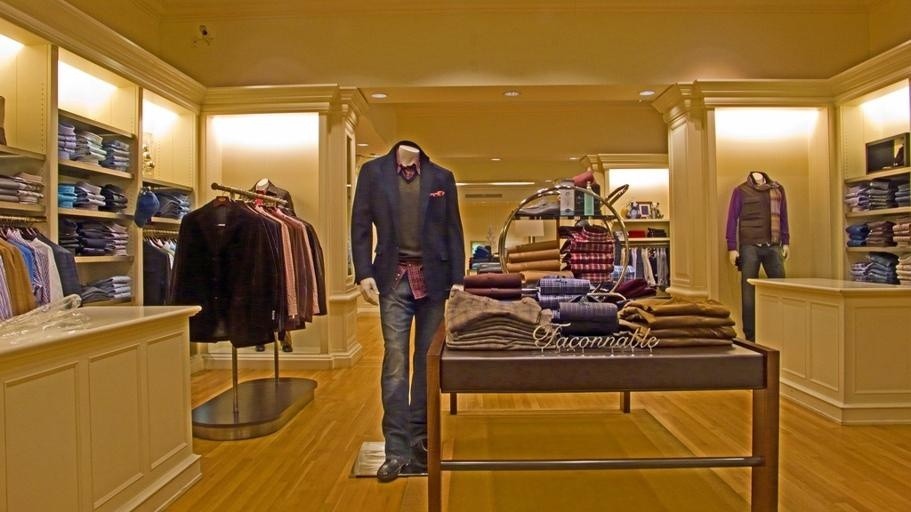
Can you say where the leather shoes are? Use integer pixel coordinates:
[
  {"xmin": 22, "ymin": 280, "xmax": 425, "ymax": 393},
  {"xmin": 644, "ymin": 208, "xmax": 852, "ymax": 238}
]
[{"xmin": 376, "ymin": 441, "xmax": 428, "ymax": 483}]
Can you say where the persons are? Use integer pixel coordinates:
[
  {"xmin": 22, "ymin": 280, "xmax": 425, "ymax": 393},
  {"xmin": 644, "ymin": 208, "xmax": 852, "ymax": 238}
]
[
  {"xmin": 350, "ymin": 139, "xmax": 467, "ymax": 482},
  {"xmin": 726, "ymin": 172, "xmax": 793, "ymax": 344}
]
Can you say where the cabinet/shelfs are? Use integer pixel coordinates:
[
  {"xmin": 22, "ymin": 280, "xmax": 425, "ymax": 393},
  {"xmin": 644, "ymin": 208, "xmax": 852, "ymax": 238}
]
[
  {"xmin": 0, "ymin": 9, "xmax": 203, "ymax": 323},
  {"xmin": 607, "ymin": 168, "xmax": 671, "ymax": 287},
  {"xmin": 840, "ymin": 72, "xmax": 911, "ymax": 286}
]
